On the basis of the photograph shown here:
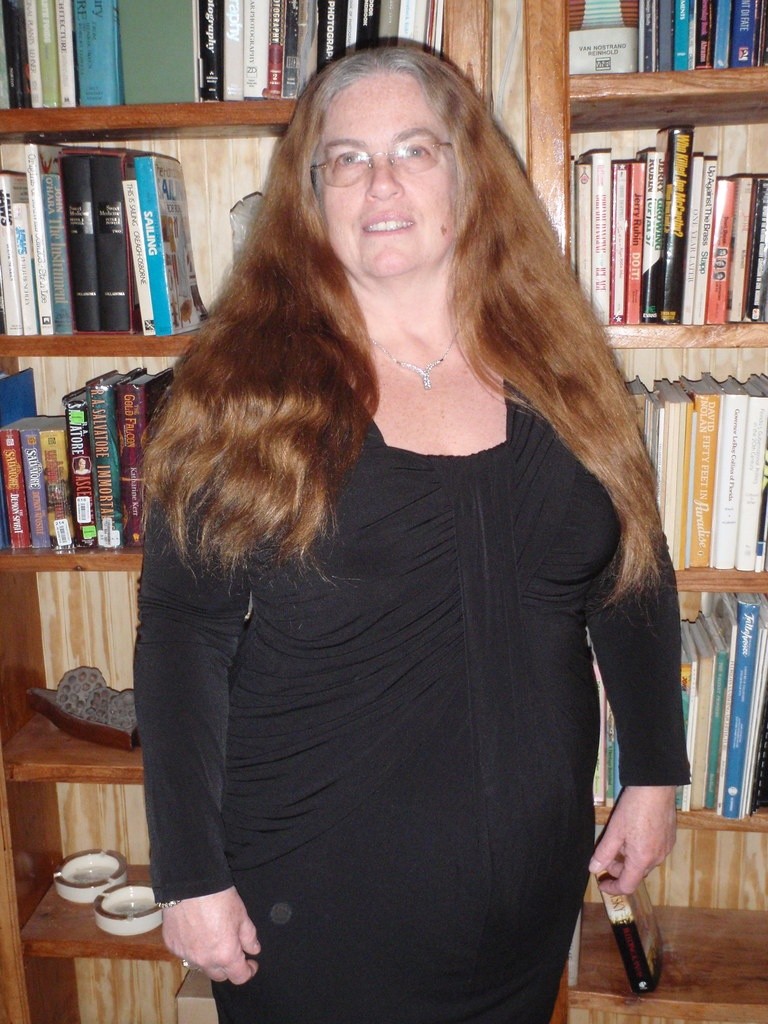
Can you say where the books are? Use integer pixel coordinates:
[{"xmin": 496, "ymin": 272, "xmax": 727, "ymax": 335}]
[
  {"xmin": 0, "ymin": 0, "xmax": 768, "ymax": 819},
  {"xmin": 595, "ymin": 867, "xmax": 660, "ymax": 994}
]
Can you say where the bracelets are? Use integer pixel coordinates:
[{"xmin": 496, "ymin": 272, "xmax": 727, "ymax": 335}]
[{"xmin": 155, "ymin": 899, "xmax": 183, "ymax": 909}]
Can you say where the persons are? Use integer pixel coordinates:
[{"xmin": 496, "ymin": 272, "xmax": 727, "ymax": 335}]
[{"xmin": 134, "ymin": 39, "xmax": 691, "ymax": 1023}]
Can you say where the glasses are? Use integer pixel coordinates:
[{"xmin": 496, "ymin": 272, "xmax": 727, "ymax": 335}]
[{"xmin": 310, "ymin": 138, "xmax": 453, "ymax": 188}]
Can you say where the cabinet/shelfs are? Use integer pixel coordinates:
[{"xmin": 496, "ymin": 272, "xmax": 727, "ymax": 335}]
[{"xmin": 0, "ymin": 0, "xmax": 768, "ymax": 1024}]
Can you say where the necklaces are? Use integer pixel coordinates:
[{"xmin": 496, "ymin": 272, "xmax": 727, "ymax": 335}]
[{"xmin": 368, "ymin": 316, "xmax": 464, "ymax": 390}]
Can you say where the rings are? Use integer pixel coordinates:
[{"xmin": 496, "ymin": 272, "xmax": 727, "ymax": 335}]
[{"xmin": 178, "ymin": 958, "xmax": 191, "ymax": 968}]
